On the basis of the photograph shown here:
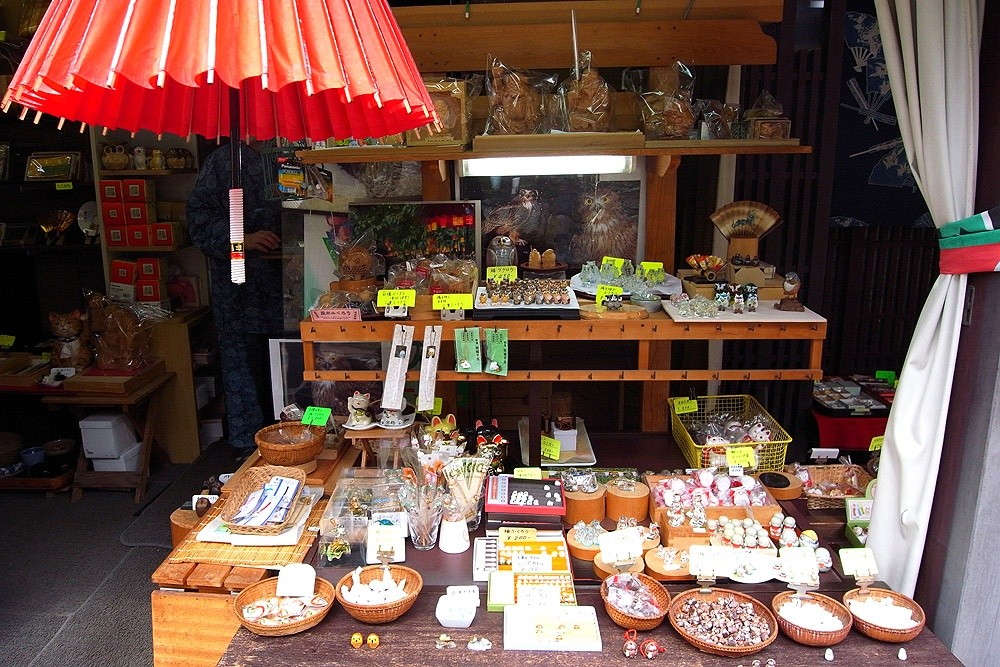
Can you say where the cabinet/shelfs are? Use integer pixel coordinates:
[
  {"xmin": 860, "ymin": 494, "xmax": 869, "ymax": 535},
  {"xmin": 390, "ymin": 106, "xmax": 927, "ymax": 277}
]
[
  {"xmin": 120, "ymin": 302, "xmax": 229, "ymax": 465},
  {"xmin": 294, "ymin": 130, "xmax": 832, "ymax": 384},
  {"xmin": 88, "ymin": 122, "xmax": 214, "ymax": 306}
]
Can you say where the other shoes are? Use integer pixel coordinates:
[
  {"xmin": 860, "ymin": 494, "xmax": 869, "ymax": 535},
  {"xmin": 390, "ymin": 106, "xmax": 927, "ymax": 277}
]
[{"xmin": 236, "ymin": 448, "xmax": 256, "ymax": 466}]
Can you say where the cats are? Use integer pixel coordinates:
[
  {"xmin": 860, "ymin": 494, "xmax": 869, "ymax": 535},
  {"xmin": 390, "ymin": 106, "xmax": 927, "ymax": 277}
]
[
  {"xmin": 347, "ymin": 391, "xmax": 373, "ymax": 428},
  {"xmin": 705, "ymin": 422, "xmax": 771, "ymax": 467},
  {"xmin": 425, "ymin": 413, "xmax": 505, "ymax": 476}
]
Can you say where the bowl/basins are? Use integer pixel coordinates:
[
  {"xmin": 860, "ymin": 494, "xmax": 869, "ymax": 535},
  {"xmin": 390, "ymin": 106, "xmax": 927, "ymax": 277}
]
[{"xmin": 21, "ymin": 439, "xmax": 74, "ymax": 466}]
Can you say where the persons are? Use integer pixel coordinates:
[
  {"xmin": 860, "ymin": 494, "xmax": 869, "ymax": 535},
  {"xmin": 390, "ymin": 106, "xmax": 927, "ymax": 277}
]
[{"xmin": 188, "ymin": 137, "xmax": 282, "ymax": 465}]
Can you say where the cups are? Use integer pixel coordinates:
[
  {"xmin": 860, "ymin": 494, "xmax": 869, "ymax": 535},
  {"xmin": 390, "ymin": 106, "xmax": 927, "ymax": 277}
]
[
  {"xmin": 446, "ymin": 484, "xmax": 486, "ymax": 531},
  {"xmin": 439, "ymin": 517, "xmax": 470, "ymax": 553},
  {"xmin": 407, "ymin": 509, "xmax": 442, "ymax": 551}
]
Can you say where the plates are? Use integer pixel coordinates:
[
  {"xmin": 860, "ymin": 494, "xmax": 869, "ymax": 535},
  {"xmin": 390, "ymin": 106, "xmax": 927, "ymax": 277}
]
[{"xmin": 77, "ymin": 201, "xmax": 100, "ymax": 236}]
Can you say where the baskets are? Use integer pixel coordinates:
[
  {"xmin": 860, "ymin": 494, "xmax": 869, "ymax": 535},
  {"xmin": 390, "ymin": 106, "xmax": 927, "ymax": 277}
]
[
  {"xmin": 843, "ymin": 588, "xmax": 925, "ymax": 643},
  {"xmin": 220, "ymin": 465, "xmax": 306, "ymax": 536},
  {"xmin": 335, "ymin": 565, "xmax": 423, "ymax": 624},
  {"xmin": 667, "ymin": 588, "xmax": 778, "ymax": 658},
  {"xmin": 771, "ymin": 590, "xmax": 853, "ymax": 646},
  {"xmin": 668, "ymin": 395, "xmax": 795, "ymax": 475},
  {"xmin": 600, "ymin": 571, "xmax": 671, "ymax": 631},
  {"xmin": 233, "ymin": 576, "xmax": 336, "ymax": 636},
  {"xmin": 255, "ymin": 421, "xmax": 327, "ymax": 466}
]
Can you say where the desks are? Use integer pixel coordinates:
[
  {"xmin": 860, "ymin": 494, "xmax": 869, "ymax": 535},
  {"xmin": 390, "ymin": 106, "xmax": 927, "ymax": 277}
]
[
  {"xmin": 41, "ymin": 370, "xmax": 176, "ymax": 504},
  {"xmin": 218, "ymin": 416, "xmax": 365, "ymax": 501},
  {"xmin": 218, "ymin": 578, "xmax": 967, "ymax": 667},
  {"xmin": 150, "ymin": 490, "xmax": 331, "ymax": 667}
]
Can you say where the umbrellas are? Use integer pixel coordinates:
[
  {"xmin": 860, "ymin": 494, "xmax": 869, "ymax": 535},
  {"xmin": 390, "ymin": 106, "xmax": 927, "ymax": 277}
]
[{"xmin": 0, "ymin": 0, "xmax": 443, "ymax": 285}]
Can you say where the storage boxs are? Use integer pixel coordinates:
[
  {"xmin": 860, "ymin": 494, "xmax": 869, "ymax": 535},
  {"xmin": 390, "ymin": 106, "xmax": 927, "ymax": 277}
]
[
  {"xmin": 98, "ymin": 178, "xmax": 187, "ymax": 303},
  {"xmin": 79, "ymin": 412, "xmax": 144, "ymax": 472}
]
